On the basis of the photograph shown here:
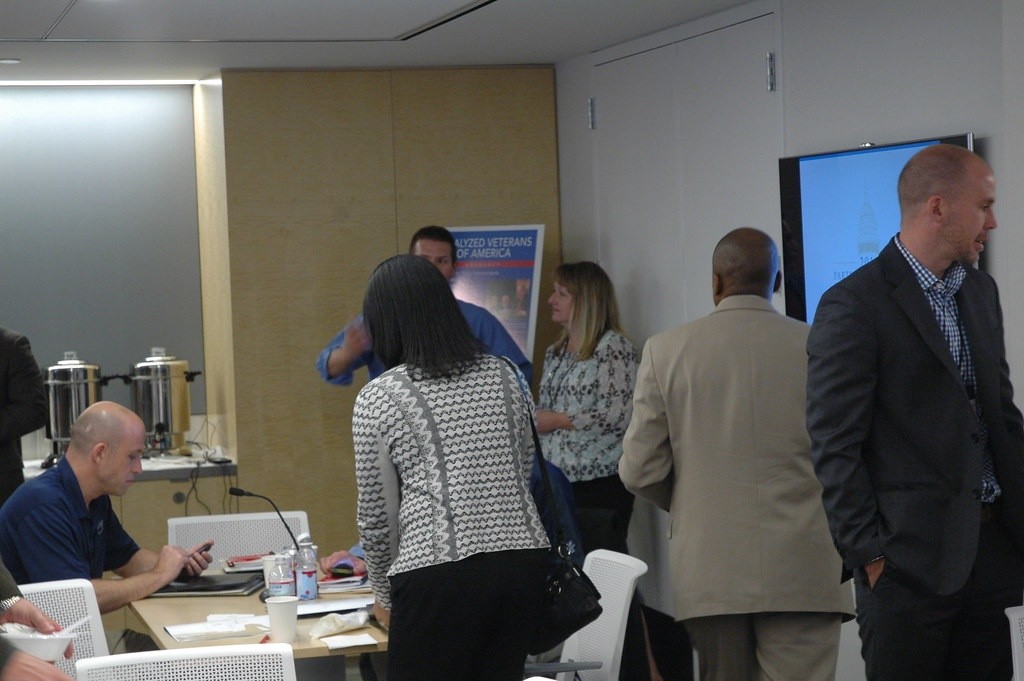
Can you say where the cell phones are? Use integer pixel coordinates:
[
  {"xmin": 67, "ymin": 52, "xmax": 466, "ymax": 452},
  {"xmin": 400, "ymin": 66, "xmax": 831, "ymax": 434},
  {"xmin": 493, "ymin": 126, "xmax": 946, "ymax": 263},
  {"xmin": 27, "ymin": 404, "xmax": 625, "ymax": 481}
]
[
  {"xmin": 330, "ymin": 568, "xmax": 354, "ymax": 574},
  {"xmin": 185, "ymin": 544, "xmax": 212, "ymax": 559}
]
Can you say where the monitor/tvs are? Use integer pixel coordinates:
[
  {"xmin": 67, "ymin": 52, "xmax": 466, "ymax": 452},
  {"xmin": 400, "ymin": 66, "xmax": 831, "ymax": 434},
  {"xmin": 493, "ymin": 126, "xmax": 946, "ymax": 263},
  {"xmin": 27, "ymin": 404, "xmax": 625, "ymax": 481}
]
[{"xmin": 778, "ymin": 132, "xmax": 974, "ymax": 325}]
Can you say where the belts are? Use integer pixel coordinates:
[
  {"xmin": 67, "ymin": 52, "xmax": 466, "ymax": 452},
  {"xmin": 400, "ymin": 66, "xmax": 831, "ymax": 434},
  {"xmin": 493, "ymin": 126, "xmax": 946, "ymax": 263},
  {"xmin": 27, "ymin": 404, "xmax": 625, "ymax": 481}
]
[{"xmin": 981, "ymin": 502, "xmax": 1000, "ymax": 525}]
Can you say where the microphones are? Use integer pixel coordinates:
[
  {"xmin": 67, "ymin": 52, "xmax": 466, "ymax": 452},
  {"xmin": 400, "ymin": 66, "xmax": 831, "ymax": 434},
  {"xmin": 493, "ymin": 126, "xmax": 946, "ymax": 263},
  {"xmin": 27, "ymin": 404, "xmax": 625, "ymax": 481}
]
[{"xmin": 228, "ymin": 486, "xmax": 300, "ymax": 554}]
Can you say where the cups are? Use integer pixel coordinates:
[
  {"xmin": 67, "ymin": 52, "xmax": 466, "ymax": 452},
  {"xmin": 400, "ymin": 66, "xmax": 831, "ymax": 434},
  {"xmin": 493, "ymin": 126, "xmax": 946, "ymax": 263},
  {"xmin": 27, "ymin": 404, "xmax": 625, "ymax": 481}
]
[
  {"xmin": 262, "ymin": 555, "xmax": 292, "ymax": 589},
  {"xmin": 265, "ymin": 596, "xmax": 299, "ymax": 643}
]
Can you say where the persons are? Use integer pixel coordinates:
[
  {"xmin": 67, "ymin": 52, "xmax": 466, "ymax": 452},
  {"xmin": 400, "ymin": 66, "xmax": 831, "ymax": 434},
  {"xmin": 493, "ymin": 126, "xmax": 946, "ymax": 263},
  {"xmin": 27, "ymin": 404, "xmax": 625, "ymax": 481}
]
[
  {"xmin": 533, "ymin": 261, "xmax": 694, "ymax": 681},
  {"xmin": 0, "ymin": 326, "xmax": 50, "ymax": 506},
  {"xmin": 319, "ymin": 543, "xmax": 367, "ymax": 578},
  {"xmin": 618, "ymin": 225, "xmax": 857, "ymax": 681},
  {"xmin": 0, "ymin": 401, "xmax": 214, "ymax": 614},
  {"xmin": 352, "ymin": 255, "xmax": 551, "ymax": 680},
  {"xmin": 807, "ymin": 144, "xmax": 1023, "ymax": 680},
  {"xmin": 0, "ymin": 557, "xmax": 77, "ymax": 681},
  {"xmin": 316, "ymin": 226, "xmax": 545, "ymax": 518}
]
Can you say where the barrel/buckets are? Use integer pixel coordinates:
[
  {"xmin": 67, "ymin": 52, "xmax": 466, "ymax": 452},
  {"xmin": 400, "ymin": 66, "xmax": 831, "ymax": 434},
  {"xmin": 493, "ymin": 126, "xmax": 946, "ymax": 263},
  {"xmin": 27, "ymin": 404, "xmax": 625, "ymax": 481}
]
[
  {"xmin": 117, "ymin": 346, "xmax": 202, "ymax": 449},
  {"xmin": 39, "ymin": 352, "xmax": 117, "ymax": 469}
]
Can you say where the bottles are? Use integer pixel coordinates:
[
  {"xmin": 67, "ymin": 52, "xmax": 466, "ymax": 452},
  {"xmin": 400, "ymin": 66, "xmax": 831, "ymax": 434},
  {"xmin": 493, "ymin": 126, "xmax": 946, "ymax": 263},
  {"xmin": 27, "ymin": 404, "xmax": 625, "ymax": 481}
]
[
  {"xmin": 269, "ymin": 557, "xmax": 295, "ymax": 597},
  {"xmin": 295, "ymin": 533, "xmax": 318, "ymax": 600}
]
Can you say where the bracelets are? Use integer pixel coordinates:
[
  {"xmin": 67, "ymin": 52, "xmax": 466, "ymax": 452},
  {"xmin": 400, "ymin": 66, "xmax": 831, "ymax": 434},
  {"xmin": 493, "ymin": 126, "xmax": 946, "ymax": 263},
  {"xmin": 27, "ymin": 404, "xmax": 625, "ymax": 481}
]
[
  {"xmin": 0, "ymin": 595, "xmax": 20, "ymax": 614},
  {"xmin": 865, "ymin": 555, "xmax": 884, "ymax": 564}
]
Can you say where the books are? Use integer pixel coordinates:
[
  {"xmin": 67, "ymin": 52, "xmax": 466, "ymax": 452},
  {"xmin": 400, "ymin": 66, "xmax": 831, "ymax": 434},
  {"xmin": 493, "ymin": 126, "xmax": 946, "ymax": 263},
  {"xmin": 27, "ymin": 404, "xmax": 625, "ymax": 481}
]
[{"xmin": 317, "ymin": 576, "xmax": 368, "ymax": 589}]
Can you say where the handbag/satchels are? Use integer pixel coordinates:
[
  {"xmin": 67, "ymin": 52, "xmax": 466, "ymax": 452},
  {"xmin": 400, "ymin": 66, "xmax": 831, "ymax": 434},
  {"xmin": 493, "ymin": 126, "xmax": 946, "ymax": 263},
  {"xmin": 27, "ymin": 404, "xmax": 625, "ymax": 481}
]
[{"xmin": 525, "ymin": 538, "xmax": 604, "ymax": 655}]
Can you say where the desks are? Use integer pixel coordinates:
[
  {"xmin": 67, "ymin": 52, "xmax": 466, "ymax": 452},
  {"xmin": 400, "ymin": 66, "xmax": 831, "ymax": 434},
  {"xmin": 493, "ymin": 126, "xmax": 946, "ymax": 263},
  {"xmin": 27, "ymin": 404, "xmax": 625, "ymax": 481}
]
[{"xmin": 131, "ymin": 584, "xmax": 389, "ymax": 681}]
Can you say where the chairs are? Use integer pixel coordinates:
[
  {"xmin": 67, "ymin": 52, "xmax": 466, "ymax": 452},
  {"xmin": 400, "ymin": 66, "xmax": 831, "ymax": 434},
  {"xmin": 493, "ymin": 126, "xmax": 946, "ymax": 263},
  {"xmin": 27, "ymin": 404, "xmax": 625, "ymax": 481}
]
[
  {"xmin": 75, "ymin": 642, "xmax": 296, "ymax": 681},
  {"xmin": 166, "ymin": 511, "xmax": 310, "ymax": 578},
  {"xmin": 522, "ymin": 547, "xmax": 650, "ymax": 681},
  {"xmin": 18, "ymin": 579, "xmax": 109, "ymax": 678}
]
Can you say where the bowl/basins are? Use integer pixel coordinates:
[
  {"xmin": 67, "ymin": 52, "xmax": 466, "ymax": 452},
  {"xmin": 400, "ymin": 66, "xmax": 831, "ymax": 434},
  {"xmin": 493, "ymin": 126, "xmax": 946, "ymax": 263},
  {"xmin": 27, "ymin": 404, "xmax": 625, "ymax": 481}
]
[{"xmin": 2, "ymin": 632, "xmax": 76, "ymax": 663}]
